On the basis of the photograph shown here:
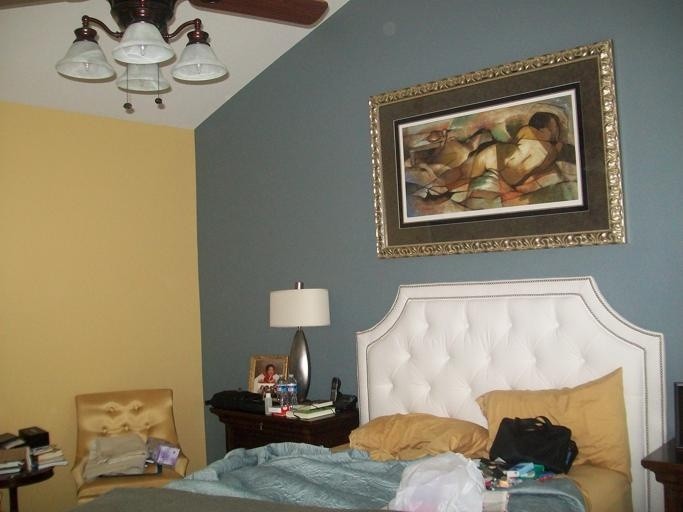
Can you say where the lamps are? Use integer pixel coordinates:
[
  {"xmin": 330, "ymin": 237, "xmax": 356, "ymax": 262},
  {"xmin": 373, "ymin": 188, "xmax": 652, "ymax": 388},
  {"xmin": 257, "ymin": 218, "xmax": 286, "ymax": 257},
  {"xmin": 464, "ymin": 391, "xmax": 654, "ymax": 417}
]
[
  {"xmin": 55, "ymin": 9, "xmax": 229, "ymax": 95},
  {"xmin": 268, "ymin": 279, "xmax": 331, "ymax": 404}
]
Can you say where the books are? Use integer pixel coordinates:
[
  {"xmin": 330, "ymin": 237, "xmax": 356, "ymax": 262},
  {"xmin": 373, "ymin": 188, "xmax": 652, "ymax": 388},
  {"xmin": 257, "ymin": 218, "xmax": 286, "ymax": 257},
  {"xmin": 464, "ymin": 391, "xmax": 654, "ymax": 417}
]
[
  {"xmin": 0, "ymin": 426, "xmax": 68, "ymax": 474},
  {"xmin": 292, "ymin": 400, "xmax": 336, "ymax": 422},
  {"xmin": 502, "ymin": 462, "xmax": 534, "ymax": 477}
]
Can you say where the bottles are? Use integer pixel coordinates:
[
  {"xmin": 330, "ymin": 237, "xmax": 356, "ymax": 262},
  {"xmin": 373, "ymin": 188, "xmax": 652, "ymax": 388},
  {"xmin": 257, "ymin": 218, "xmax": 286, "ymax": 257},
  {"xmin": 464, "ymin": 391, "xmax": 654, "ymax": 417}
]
[
  {"xmin": 276, "ymin": 373, "xmax": 297, "ymax": 413},
  {"xmin": 264, "ymin": 393, "xmax": 272, "ymax": 416}
]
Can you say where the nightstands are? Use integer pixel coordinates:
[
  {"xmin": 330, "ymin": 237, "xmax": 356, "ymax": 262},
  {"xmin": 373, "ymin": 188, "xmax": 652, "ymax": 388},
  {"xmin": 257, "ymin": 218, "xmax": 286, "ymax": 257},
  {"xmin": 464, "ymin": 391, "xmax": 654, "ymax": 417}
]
[
  {"xmin": 641, "ymin": 440, "xmax": 682, "ymax": 510},
  {"xmin": 208, "ymin": 407, "xmax": 358, "ymax": 456}
]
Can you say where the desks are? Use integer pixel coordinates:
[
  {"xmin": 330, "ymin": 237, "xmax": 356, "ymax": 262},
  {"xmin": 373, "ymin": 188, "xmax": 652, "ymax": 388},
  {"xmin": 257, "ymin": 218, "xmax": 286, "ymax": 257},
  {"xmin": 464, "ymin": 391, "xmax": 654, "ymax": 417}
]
[{"xmin": 1, "ymin": 468, "xmax": 55, "ymax": 511}]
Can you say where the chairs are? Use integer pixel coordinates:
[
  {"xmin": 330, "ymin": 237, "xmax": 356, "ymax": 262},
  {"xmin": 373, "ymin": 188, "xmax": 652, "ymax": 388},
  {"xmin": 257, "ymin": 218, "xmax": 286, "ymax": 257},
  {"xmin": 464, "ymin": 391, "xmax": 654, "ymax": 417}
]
[{"xmin": 69, "ymin": 388, "xmax": 189, "ymax": 505}]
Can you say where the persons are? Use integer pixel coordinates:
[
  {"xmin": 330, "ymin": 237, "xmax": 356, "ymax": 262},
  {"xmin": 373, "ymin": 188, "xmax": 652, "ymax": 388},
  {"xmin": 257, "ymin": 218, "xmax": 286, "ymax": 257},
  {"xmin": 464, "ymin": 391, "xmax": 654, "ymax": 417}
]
[{"xmin": 254, "ymin": 365, "xmax": 275, "ymax": 383}]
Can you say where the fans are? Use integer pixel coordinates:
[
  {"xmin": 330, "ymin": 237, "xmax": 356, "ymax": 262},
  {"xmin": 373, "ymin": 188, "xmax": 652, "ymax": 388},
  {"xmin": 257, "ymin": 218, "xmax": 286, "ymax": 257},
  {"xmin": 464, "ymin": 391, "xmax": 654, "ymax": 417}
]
[{"xmin": 1, "ymin": 1, "xmax": 329, "ymax": 26}]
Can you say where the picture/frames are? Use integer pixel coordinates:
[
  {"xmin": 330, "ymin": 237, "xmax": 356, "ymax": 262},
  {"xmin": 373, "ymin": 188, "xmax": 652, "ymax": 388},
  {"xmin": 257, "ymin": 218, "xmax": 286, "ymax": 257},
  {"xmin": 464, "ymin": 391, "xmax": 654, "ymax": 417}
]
[
  {"xmin": 369, "ymin": 40, "xmax": 626, "ymax": 260},
  {"xmin": 246, "ymin": 354, "xmax": 290, "ymax": 392}
]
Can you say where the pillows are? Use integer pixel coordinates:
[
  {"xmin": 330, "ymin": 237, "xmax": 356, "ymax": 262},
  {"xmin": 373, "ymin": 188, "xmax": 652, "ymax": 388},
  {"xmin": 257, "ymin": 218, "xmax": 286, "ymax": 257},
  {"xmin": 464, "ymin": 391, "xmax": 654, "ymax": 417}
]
[{"xmin": 349, "ymin": 366, "xmax": 633, "ymax": 480}]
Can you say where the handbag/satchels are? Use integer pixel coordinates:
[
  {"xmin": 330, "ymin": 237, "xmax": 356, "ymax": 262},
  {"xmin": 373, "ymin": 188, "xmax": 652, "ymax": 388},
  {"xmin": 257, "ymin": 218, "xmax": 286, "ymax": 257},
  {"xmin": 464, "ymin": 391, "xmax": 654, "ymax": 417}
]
[
  {"xmin": 489, "ymin": 416, "xmax": 578, "ymax": 474},
  {"xmin": 204, "ymin": 390, "xmax": 280, "ymax": 414}
]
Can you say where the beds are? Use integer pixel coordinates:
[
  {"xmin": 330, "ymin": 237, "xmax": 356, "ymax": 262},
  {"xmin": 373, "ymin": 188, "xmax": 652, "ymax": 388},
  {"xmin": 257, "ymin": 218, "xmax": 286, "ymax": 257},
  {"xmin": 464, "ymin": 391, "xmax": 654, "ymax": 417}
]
[{"xmin": 80, "ymin": 274, "xmax": 669, "ymax": 512}]
